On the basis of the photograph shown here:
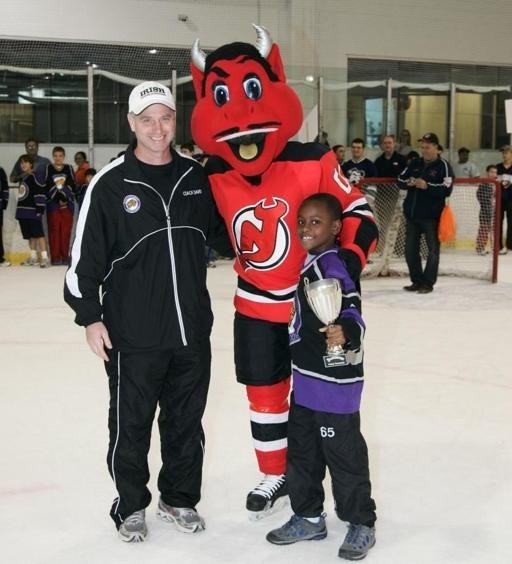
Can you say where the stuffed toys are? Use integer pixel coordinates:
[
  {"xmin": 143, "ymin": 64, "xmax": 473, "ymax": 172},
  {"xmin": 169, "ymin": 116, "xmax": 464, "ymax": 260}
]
[{"xmin": 189, "ymin": 20, "xmax": 379, "ymax": 520}]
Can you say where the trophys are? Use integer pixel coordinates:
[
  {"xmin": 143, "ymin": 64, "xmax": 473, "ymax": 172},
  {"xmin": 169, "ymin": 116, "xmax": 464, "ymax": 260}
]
[{"xmin": 304, "ymin": 276, "xmax": 348, "ymax": 367}]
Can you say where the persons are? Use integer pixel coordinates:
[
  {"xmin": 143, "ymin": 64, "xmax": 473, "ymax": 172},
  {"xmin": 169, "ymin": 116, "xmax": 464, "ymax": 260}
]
[
  {"xmin": 2, "ymin": 130, "xmax": 511, "ymax": 268},
  {"xmin": 395, "ymin": 132, "xmax": 454, "ymax": 293},
  {"xmin": 63, "ymin": 80, "xmax": 237, "ymax": 540},
  {"xmin": 266, "ymin": 192, "xmax": 376, "ymax": 559}
]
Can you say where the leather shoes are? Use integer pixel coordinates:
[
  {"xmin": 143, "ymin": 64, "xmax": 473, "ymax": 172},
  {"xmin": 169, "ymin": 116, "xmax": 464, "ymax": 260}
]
[{"xmin": 405, "ymin": 283, "xmax": 432, "ymax": 294}]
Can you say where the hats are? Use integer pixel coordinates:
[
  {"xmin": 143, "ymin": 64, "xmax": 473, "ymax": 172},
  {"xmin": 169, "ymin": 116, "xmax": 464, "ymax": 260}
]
[
  {"xmin": 458, "ymin": 147, "xmax": 470, "ymax": 153},
  {"xmin": 418, "ymin": 133, "xmax": 439, "ymax": 144},
  {"xmin": 500, "ymin": 145, "xmax": 512, "ymax": 151},
  {"xmin": 128, "ymin": 82, "xmax": 177, "ymax": 115}
]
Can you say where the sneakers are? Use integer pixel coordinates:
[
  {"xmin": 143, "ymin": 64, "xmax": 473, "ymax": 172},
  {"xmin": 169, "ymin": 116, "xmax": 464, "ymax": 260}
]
[
  {"xmin": 478, "ymin": 248, "xmax": 508, "ymax": 256},
  {"xmin": 1, "ymin": 258, "xmax": 69, "ymax": 267},
  {"xmin": 116, "ymin": 496, "xmax": 206, "ymax": 544},
  {"xmin": 266, "ymin": 515, "xmax": 377, "ymax": 561}
]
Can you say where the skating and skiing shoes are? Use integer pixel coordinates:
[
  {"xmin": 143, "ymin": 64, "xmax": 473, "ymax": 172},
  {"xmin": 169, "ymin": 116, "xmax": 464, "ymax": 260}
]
[{"xmin": 246, "ymin": 473, "xmax": 291, "ymax": 522}]
